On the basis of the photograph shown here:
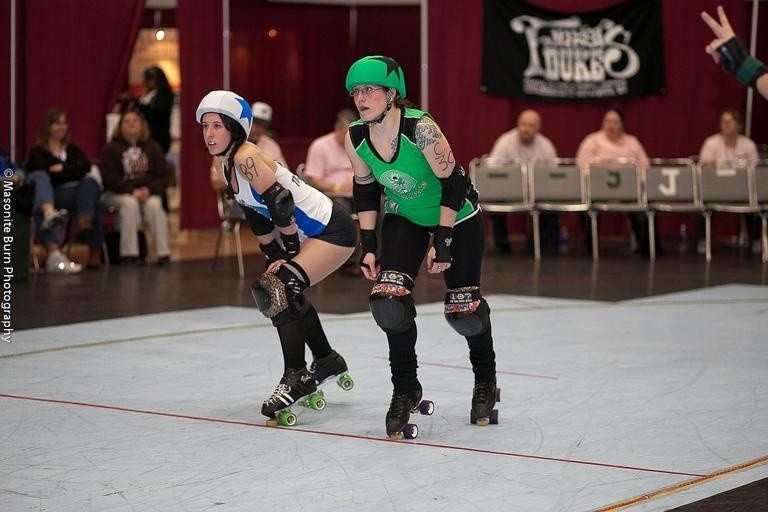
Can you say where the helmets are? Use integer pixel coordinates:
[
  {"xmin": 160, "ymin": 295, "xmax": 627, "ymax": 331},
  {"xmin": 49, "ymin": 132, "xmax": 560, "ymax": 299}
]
[
  {"xmin": 345, "ymin": 55, "xmax": 407, "ymax": 124},
  {"xmin": 196, "ymin": 90, "xmax": 253, "ymax": 156}
]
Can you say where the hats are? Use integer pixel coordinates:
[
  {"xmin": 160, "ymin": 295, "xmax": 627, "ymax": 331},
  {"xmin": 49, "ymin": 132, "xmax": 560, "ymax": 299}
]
[{"xmin": 249, "ymin": 102, "xmax": 273, "ymax": 123}]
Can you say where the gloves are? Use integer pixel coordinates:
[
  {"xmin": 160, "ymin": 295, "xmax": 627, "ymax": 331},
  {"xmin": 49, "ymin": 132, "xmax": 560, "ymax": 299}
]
[
  {"xmin": 275, "ymin": 230, "xmax": 301, "ymax": 262},
  {"xmin": 256, "ymin": 239, "xmax": 284, "ymax": 273},
  {"xmin": 358, "ymin": 229, "xmax": 381, "ymax": 269},
  {"xmin": 431, "ymin": 224, "xmax": 455, "ymax": 264}
]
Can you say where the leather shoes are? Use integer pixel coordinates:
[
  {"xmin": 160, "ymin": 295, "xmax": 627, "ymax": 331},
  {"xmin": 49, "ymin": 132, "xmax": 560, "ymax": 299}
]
[
  {"xmin": 122, "ymin": 255, "xmax": 139, "ymax": 266},
  {"xmin": 157, "ymin": 256, "xmax": 175, "ymax": 270},
  {"xmin": 487, "ymin": 242, "xmax": 512, "ymax": 257}
]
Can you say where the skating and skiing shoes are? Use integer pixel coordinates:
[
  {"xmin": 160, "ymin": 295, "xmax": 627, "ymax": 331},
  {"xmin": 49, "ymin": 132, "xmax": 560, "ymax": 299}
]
[
  {"xmin": 310, "ymin": 351, "xmax": 355, "ymax": 392},
  {"xmin": 469, "ymin": 368, "xmax": 503, "ymax": 425},
  {"xmin": 260, "ymin": 366, "xmax": 326, "ymax": 426},
  {"xmin": 384, "ymin": 377, "xmax": 435, "ymax": 439}
]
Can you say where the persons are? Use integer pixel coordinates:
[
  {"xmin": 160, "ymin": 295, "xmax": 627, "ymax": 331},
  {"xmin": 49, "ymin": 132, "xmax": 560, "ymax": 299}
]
[
  {"xmin": 693, "ymin": 4, "xmax": 768, "ymax": 101},
  {"xmin": 25, "ymin": 106, "xmax": 104, "ymax": 274},
  {"xmin": 0, "ymin": 147, "xmax": 84, "ymax": 275},
  {"xmin": 211, "ymin": 99, "xmax": 293, "ymax": 222},
  {"xmin": 695, "ymin": 107, "xmax": 762, "ymax": 259},
  {"xmin": 132, "ymin": 66, "xmax": 177, "ymax": 214},
  {"xmin": 342, "ymin": 52, "xmax": 504, "ymax": 443},
  {"xmin": 483, "ymin": 107, "xmax": 561, "ymax": 261},
  {"xmin": 300, "ymin": 106, "xmax": 357, "ymax": 280},
  {"xmin": 573, "ymin": 107, "xmax": 662, "ymax": 259},
  {"xmin": 193, "ymin": 87, "xmax": 361, "ymax": 429},
  {"xmin": 99, "ymin": 106, "xmax": 177, "ymax": 270}
]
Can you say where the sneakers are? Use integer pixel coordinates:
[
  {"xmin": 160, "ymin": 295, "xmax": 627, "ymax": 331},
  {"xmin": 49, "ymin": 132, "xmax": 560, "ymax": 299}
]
[{"xmin": 44, "ymin": 254, "xmax": 82, "ymax": 274}]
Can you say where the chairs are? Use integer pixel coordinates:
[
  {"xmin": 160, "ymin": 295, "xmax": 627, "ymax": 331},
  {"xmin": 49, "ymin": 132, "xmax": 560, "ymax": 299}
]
[
  {"xmin": 212, "ymin": 187, "xmax": 250, "ymax": 277},
  {"xmin": 21, "ymin": 158, "xmax": 102, "ymax": 274},
  {"xmin": 469, "ymin": 155, "xmax": 541, "ymax": 265},
  {"xmin": 527, "ymin": 156, "xmax": 598, "ymax": 264},
  {"xmin": 638, "ymin": 158, "xmax": 711, "ymax": 263},
  {"xmin": 296, "ymin": 163, "xmax": 356, "ymax": 278},
  {"xmin": 584, "ymin": 157, "xmax": 655, "ymax": 264},
  {"xmin": 696, "ymin": 158, "xmax": 767, "ymax": 262}
]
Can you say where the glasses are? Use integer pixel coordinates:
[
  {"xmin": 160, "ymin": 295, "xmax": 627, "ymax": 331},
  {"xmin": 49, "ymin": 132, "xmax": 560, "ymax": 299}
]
[{"xmin": 350, "ymin": 87, "xmax": 382, "ymax": 97}]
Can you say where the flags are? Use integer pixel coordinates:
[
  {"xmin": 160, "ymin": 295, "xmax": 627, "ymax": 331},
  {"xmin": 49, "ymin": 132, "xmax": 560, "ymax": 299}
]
[{"xmin": 479, "ymin": 0, "xmax": 670, "ymax": 103}]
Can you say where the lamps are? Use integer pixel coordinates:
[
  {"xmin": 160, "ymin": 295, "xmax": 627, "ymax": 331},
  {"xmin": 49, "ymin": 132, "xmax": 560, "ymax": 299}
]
[{"xmin": 155, "ymin": 28, "xmax": 167, "ymax": 42}]
